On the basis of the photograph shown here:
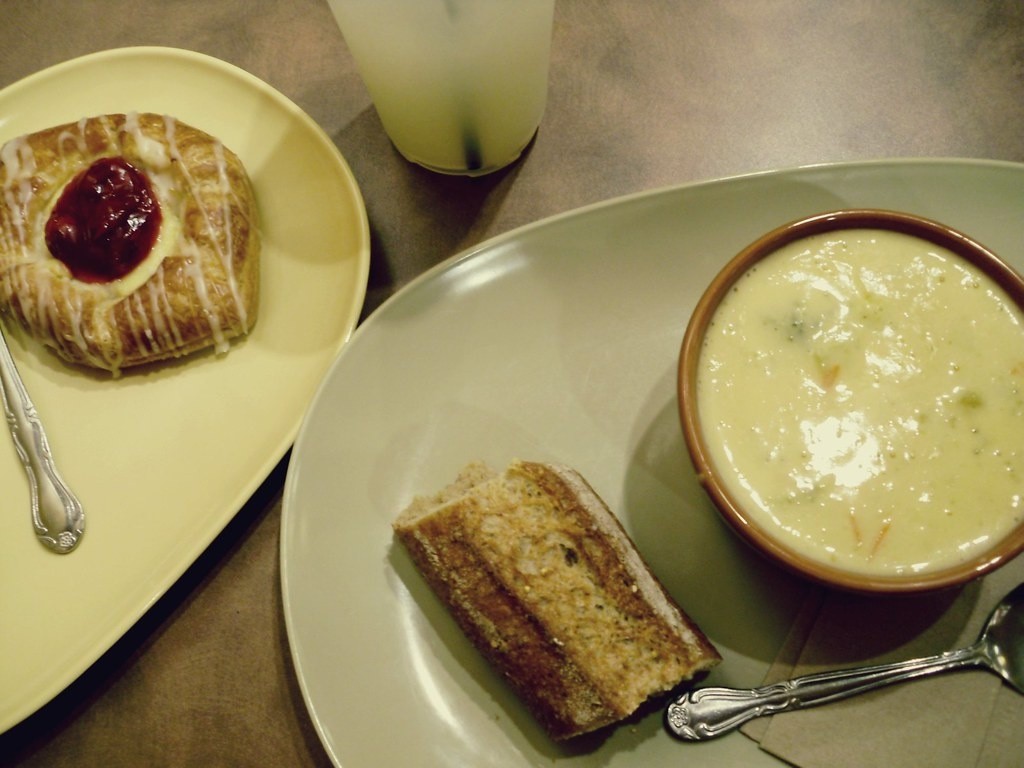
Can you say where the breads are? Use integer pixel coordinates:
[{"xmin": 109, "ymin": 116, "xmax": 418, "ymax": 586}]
[
  {"xmin": 392, "ymin": 451, "xmax": 721, "ymax": 743},
  {"xmin": 0, "ymin": 114, "xmax": 259, "ymax": 370}
]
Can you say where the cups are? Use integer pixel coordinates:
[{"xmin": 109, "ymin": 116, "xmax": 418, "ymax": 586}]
[{"xmin": 328, "ymin": 0, "xmax": 554, "ymax": 176}]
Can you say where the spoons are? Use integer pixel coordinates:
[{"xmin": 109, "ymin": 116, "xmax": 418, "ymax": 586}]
[{"xmin": 665, "ymin": 583, "xmax": 1022, "ymax": 740}]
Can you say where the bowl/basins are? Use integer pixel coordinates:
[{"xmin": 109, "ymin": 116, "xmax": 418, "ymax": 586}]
[{"xmin": 678, "ymin": 209, "xmax": 1023, "ymax": 594}]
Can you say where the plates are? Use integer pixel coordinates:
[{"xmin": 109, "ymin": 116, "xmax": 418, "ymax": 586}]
[
  {"xmin": 279, "ymin": 158, "xmax": 1023, "ymax": 767},
  {"xmin": 0, "ymin": 47, "xmax": 368, "ymax": 732}
]
